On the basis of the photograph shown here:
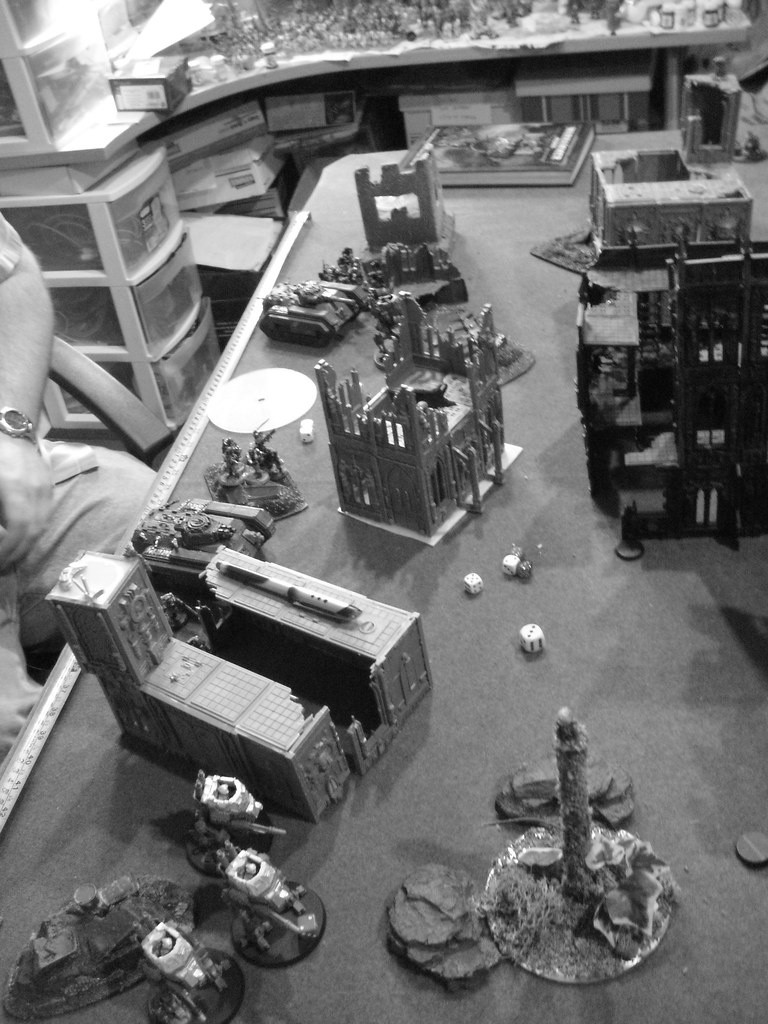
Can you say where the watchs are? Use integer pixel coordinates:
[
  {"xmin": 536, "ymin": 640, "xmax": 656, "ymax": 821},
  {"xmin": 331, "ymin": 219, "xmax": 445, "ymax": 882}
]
[{"xmin": 0, "ymin": 406, "xmax": 37, "ymax": 444}]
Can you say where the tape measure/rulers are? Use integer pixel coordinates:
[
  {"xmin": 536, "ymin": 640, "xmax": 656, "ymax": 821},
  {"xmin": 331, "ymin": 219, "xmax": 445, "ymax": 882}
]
[{"xmin": 0, "ymin": 205, "xmax": 311, "ymax": 838}]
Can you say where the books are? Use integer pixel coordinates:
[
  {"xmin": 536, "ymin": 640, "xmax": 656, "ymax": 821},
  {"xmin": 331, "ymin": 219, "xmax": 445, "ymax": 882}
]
[{"xmin": 398, "ymin": 122, "xmax": 597, "ymax": 188}]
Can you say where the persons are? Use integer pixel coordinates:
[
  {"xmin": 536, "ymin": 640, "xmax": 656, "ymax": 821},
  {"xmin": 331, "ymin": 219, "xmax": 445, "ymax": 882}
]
[
  {"xmin": 0, "ymin": 210, "xmax": 157, "ymax": 767},
  {"xmin": 222, "ymin": 429, "xmax": 278, "ymax": 477}
]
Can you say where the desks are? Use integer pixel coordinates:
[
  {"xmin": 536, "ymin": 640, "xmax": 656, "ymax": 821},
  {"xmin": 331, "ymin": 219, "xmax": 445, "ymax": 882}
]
[
  {"xmin": 0, "ymin": 0, "xmax": 752, "ymax": 165},
  {"xmin": 0, "ymin": 121, "xmax": 768, "ymax": 1024}
]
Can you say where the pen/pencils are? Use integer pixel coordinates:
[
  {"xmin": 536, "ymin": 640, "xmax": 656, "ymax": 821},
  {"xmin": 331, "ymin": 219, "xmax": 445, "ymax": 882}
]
[{"xmin": 215, "ymin": 557, "xmax": 363, "ymax": 624}]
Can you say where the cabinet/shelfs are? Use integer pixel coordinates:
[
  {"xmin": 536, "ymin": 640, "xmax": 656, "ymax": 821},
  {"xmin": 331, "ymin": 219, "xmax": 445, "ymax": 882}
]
[{"xmin": 0, "ymin": 141, "xmax": 222, "ymax": 451}]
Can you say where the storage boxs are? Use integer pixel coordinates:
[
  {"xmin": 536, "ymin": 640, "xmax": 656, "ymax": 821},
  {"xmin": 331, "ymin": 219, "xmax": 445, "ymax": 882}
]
[
  {"xmin": 110, "ymin": 52, "xmax": 189, "ymax": 112},
  {"xmin": 1, "ymin": 139, "xmax": 140, "ymax": 195},
  {"xmin": 397, "ymin": 59, "xmax": 518, "ymax": 149},
  {"xmin": 513, "ymin": 47, "xmax": 657, "ymax": 130},
  {"xmin": 144, "ymin": 76, "xmax": 393, "ymax": 352}
]
